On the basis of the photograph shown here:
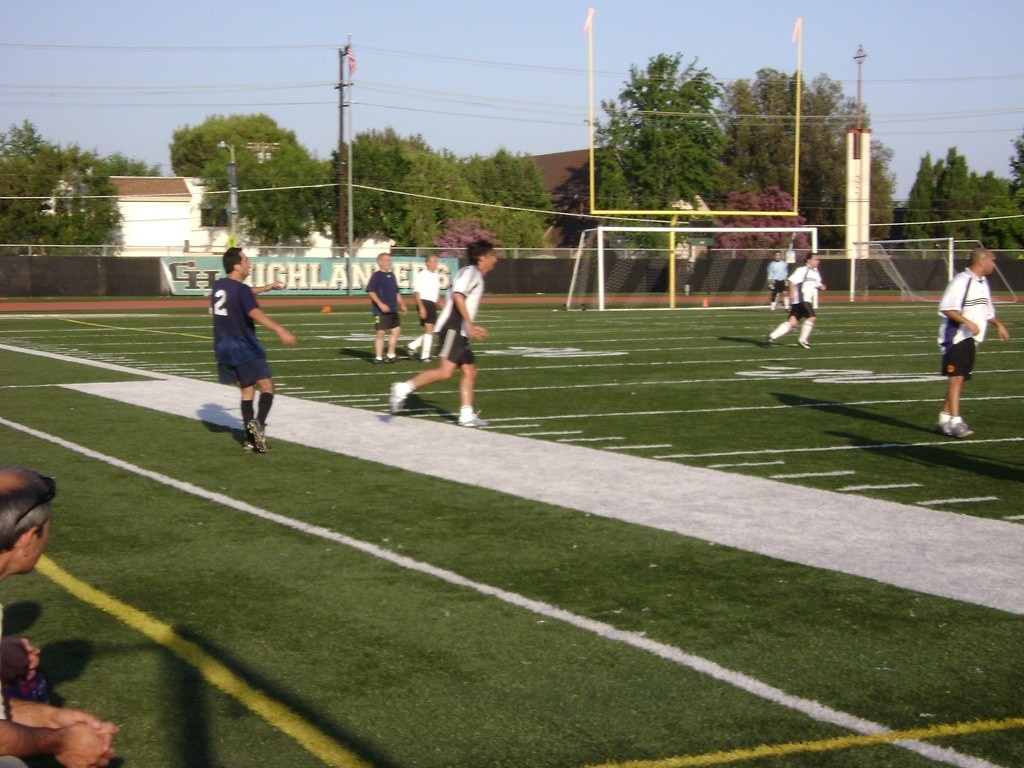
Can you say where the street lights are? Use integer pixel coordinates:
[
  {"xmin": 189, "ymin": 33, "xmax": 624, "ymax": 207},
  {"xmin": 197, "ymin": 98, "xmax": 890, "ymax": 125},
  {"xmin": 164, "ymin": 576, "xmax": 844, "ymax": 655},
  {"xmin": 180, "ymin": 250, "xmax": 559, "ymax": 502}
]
[{"xmin": 217, "ymin": 141, "xmax": 238, "ymax": 247}]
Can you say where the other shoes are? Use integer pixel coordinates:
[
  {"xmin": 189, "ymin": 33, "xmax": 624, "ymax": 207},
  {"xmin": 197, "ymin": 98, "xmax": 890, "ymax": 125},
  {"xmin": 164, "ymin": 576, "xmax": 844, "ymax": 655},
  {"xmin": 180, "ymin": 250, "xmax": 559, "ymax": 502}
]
[
  {"xmin": 796, "ymin": 339, "xmax": 812, "ymax": 349},
  {"xmin": 373, "ymin": 358, "xmax": 385, "ymax": 364},
  {"xmin": 766, "ymin": 334, "xmax": 775, "ymax": 344},
  {"xmin": 420, "ymin": 358, "xmax": 432, "ymax": 363},
  {"xmin": 405, "ymin": 346, "xmax": 414, "ymax": 361},
  {"xmin": 386, "ymin": 356, "xmax": 399, "ymax": 362}
]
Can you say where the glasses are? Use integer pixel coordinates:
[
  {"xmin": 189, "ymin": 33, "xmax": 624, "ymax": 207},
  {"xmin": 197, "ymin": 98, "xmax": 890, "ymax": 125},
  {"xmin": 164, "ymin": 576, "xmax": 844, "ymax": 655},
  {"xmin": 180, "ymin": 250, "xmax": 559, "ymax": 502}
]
[{"xmin": 15, "ymin": 474, "xmax": 56, "ymax": 526}]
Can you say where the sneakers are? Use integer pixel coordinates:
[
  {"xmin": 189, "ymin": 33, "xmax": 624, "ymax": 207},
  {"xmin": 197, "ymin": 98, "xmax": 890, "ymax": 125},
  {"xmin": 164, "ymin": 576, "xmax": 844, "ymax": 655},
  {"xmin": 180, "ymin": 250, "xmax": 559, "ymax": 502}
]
[
  {"xmin": 243, "ymin": 441, "xmax": 275, "ymax": 453},
  {"xmin": 937, "ymin": 422, "xmax": 953, "ymax": 436},
  {"xmin": 457, "ymin": 409, "xmax": 488, "ymax": 426},
  {"xmin": 389, "ymin": 382, "xmax": 407, "ymax": 415},
  {"xmin": 247, "ymin": 419, "xmax": 270, "ymax": 452},
  {"xmin": 950, "ymin": 419, "xmax": 974, "ymax": 438}
]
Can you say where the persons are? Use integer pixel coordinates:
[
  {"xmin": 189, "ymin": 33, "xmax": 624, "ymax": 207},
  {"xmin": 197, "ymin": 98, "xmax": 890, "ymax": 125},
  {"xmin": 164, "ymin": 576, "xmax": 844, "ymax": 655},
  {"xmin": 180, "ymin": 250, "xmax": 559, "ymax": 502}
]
[
  {"xmin": 389, "ymin": 240, "xmax": 498, "ymax": 426},
  {"xmin": 210, "ymin": 248, "xmax": 297, "ymax": 452},
  {"xmin": 767, "ymin": 251, "xmax": 792, "ymax": 312},
  {"xmin": 0, "ymin": 467, "xmax": 121, "ymax": 768},
  {"xmin": 366, "ymin": 252, "xmax": 410, "ymax": 363},
  {"xmin": 403, "ymin": 253, "xmax": 445, "ymax": 364},
  {"xmin": 766, "ymin": 253, "xmax": 826, "ymax": 349},
  {"xmin": 936, "ymin": 248, "xmax": 1010, "ymax": 438}
]
